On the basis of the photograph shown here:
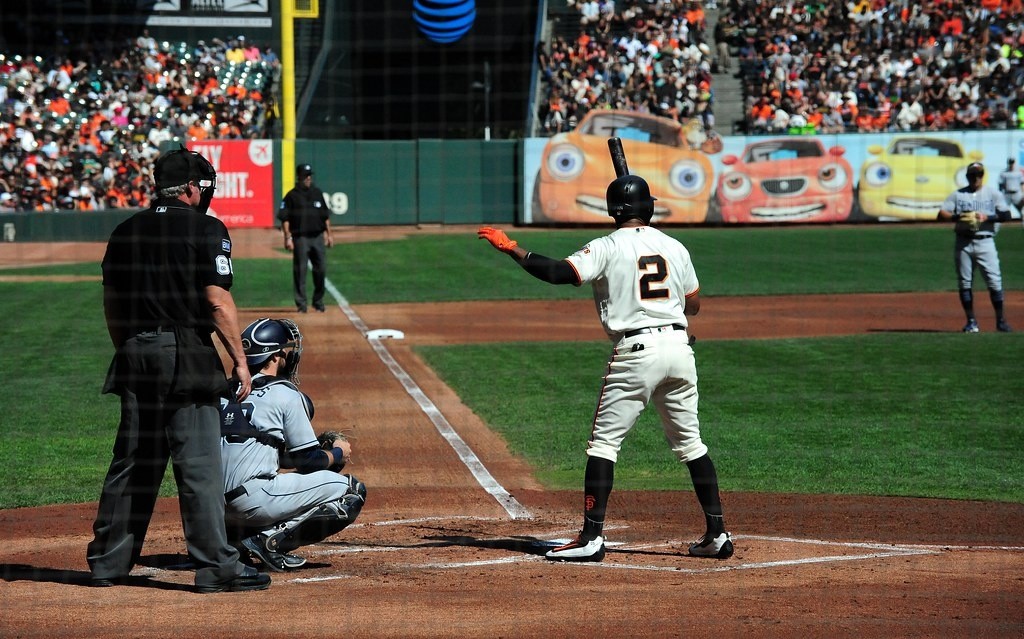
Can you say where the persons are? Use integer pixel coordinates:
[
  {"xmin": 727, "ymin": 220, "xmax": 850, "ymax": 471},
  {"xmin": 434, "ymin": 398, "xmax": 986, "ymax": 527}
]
[
  {"xmin": 1000, "ymin": 157, "xmax": 1024, "ymax": 211},
  {"xmin": 277, "ymin": 163, "xmax": 335, "ymax": 313},
  {"xmin": 87, "ymin": 144, "xmax": 271, "ymax": 594},
  {"xmin": 0, "ymin": 27, "xmax": 282, "ymax": 211},
  {"xmin": 218, "ymin": 318, "xmax": 366, "ymax": 573},
  {"xmin": 477, "ymin": 174, "xmax": 736, "ymax": 560},
  {"xmin": 536, "ymin": 0, "xmax": 1024, "ymax": 137},
  {"xmin": 941, "ymin": 162, "xmax": 1013, "ymax": 333}
]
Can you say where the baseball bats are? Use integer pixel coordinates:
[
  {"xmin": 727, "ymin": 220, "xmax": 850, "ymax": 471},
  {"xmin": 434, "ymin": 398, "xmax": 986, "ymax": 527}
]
[{"xmin": 608, "ymin": 137, "xmax": 629, "ymax": 177}]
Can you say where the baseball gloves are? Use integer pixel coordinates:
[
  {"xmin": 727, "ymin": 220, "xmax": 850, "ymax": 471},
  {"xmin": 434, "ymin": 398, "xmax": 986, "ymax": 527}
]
[
  {"xmin": 316, "ymin": 431, "xmax": 349, "ymax": 474},
  {"xmin": 959, "ymin": 211, "xmax": 982, "ymax": 233}
]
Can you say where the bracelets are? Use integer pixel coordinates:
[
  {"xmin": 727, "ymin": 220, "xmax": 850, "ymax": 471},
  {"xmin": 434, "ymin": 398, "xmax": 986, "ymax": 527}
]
[
  {"xmin": 285, "ymin": 236, "xmax": 293, "ymax": 239},
  {"xmin": 331, "ymin": 446, "xmax": 343, "ymax": 467}
]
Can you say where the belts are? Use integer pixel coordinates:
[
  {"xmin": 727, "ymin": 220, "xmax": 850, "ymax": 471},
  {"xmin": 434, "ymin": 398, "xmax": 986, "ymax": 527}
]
[
  {"xmin": 625, "ymin": 324, "xmax": 685, "ymax": 339},
  {"xmin": 972, "ymin": 235, "xmax": 991, "ymax": 239},
  {"xmin": 224, "ymin": 486, "xmax": 248, "ymax": 504},
  {"xmin": 127, "ymin": 324, "xmax": 176, "ymax": 332}
]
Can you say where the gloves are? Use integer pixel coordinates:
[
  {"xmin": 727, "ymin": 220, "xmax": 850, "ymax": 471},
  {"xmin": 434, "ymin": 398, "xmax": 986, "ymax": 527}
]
[{"xmin": 477, "ymin": 226, "xmax": 517, "ymax": 254}]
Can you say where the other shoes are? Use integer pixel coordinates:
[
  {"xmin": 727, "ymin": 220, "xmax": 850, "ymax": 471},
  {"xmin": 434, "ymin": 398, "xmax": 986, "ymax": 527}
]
[
  {"xmin": 315, "ymin": 305, "xmax": 325, "ymax": 312},
  {"xmin": 196, "ymin": 565, "xmax": 271, "ymax": 592},
  {"xmin": 997, "ymin": 322, "xmax": 1011, "ymax": 332},
  {"xmin": 241, "ymin": 533, "xmax": 291, "ymax": 573},
  {"xmin": 92, "ymin": 578, "xmax": 115, "ymax": 587},
  {"xmin": 963, "ymin": 322, "xmax": 979, "ymax": 334},
  {"xmin": 298, "ymin": 306, "xmax": 307, "ymax": 314}
]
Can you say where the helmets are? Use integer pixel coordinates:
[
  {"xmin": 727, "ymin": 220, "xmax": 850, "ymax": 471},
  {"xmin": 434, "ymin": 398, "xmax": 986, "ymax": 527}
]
[
  {"xmin": 240, "ymin": 318, "xmax": 304, "ymax": 385},
  {"xmin": 606, "ymin": 176, "xmax": 656, "ymax": 217}
]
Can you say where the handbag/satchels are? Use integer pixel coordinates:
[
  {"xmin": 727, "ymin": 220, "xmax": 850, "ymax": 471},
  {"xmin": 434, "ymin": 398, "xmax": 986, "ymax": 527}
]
[
  {"xmin": 170, "ymin": 325, "xmax": 233, "ymax": 401},
  {"xmin": 102, "ymin": 351, "xmax": 121, "ymax": 397}
]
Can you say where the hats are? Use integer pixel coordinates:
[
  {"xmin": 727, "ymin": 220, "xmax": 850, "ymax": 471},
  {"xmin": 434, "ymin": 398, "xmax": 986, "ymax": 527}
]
[
  {"xmin": 297, "ymin": 164, "xmax": 313, "ymax": 178},
  {"xmin": 155, "ymin": 143, "xmax": 217, "ymax": 193},
  {"xmin": 967, "ymin": 162, "xmax": 985, "ymax": 175}
]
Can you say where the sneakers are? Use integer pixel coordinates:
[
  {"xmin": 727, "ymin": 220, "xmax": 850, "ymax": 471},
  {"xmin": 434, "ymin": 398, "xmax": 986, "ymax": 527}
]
[
  {"xmin": 689, "ymin": 532, "xmax": 734, "ymax": 559},
  {"xmin": 544, "ymin": 533, "xmax": 607, "ymax": 563}
]
